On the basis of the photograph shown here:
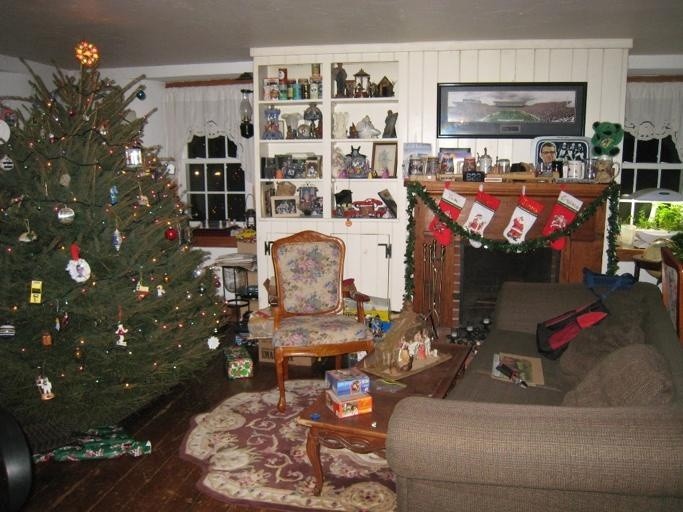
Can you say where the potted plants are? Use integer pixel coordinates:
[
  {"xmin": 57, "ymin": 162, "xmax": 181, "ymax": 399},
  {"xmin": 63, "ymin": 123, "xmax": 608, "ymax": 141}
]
[{"xmin": 637, "ymin": 203, "xmax": 682, "ymax": 242}]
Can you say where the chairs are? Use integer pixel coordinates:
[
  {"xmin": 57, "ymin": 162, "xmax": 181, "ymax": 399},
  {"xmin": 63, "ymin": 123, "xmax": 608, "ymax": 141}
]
[
  {"xmin": 268, "ymin": 230, "xmax": 377, "ymax": 412},
  {"xmin": 659, "ymin": 246, "xmax": 681, "ymax": 346}
]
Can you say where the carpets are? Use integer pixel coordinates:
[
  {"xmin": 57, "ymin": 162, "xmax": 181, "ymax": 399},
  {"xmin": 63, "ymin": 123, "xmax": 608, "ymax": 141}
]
[{"xmin": 177, "ymin": 375, "xmax": 403, "ymax": 512}]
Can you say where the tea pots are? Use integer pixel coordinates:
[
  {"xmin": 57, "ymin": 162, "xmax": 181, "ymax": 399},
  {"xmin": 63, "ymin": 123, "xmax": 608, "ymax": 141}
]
[{"xmin": 476, "ymin": 148, "xmax": 492, "ymax": 175}]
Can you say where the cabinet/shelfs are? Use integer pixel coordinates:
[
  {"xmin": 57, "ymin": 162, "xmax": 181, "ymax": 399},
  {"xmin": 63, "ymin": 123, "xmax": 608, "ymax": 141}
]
[{"xmin": 249, "ymin": 43, "xmax": 405, "ymax": 315}]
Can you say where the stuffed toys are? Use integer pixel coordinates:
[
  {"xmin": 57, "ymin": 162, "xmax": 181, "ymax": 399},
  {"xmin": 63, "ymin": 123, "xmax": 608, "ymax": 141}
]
[{"xmin": 591, "ymin": 120, "xmax": 623, "ymax": 157}]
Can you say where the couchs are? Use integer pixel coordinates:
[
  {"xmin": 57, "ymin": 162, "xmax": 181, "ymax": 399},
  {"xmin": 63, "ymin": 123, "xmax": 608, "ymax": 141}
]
[{"xmin": 384, "ymin": 278, "xmax": 682, "ymax": 512}]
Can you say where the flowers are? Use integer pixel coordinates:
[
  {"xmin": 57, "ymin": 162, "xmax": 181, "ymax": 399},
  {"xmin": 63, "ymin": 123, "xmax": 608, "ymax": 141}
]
[{"xmin": 235, "ymin": 227, "xmax": 257, "ymax": 243}]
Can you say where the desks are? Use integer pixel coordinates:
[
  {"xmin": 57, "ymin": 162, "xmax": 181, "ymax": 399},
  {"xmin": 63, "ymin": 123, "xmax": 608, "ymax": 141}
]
[
  {"xmin": 212, "ymin": 262, "xmax": 251, "ymax": 313},
  {"xmin": 632, "ymin": 255, "xmax": 664, "ymax": 290}
]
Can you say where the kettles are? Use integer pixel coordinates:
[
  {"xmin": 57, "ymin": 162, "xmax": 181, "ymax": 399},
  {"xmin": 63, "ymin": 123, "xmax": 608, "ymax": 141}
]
[{"xmin": 592, "ymin": 154, "xmax": 619, "ymax": 184}]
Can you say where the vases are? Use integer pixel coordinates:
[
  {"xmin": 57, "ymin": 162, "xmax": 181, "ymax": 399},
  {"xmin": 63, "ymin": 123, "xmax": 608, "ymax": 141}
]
[{"xmin": 234, "ymin": 240, "xmax": 257, "ymax": 311}]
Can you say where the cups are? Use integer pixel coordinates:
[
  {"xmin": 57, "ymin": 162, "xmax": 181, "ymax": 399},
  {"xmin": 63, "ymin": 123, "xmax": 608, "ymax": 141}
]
[
  {"xmin": 408, "ymin": 157, "xmax": 423, "ymax": 178},
  {"xmin": 425, "ymin": 156, "xmax": 438, "ymax": 176},
  {"xmin": 438, "ymin": 154, "xmax": 455, "ymax": 175},
  {"xmin": 461, "ymin": 157, "xmax": 475, "ymax": 174},
  {"xmin": 495, "ymin": 158, "xmax": 510, "ymax": 183},
  {"xmin": 566, "ymin": 158, "xmax": 585, "ymax": 181}
]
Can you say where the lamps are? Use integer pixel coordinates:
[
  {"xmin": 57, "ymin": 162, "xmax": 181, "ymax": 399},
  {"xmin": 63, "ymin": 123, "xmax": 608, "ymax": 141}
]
[{"xmin": 237, "ymin": 88, "xmax": 255, "ymax": 139}]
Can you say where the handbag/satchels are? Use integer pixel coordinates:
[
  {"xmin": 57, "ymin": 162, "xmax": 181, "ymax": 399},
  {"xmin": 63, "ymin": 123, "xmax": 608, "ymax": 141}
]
[
  {"xmin": 537, "ymin": 298, "xmax": 611, "ymax": 359},
  {"xmin": 583, "ymin": 267, "xmax": 635, "ymax": 300}
]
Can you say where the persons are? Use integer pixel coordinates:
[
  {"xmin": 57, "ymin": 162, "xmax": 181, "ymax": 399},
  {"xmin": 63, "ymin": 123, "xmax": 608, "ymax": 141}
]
[
  {"xmin": 76, "ymin": 264, "xmax": 85, "ymax": 279},
  {"xmin": 276, "ymin": 200, "xmax": 295, "ymax": 215},
  {"xmin": 307, "ymin": 164, "xmax": 316, "ymax": 177},
  {"xmin": 539, "ymin": 141, "xmax": 584, "ymax": 172},
  {"xmin": 382, "ymin": 110, "xmax": 398, "ymax": 138}
]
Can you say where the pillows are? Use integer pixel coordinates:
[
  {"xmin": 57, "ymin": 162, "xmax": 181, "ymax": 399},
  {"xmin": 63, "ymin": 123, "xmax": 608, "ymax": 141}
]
[{"xmin": 559, "ymin": 280, "xmax": 677, "ymax": 415}]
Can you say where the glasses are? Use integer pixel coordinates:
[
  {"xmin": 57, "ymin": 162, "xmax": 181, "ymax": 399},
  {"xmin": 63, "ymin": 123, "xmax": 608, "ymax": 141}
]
[{"xmin": 542, "ymin": 150, "xmax": 555, "ymax": 154}]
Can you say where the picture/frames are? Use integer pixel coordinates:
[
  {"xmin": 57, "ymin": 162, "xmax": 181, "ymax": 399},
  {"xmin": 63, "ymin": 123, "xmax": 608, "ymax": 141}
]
[
  {"xmin": 532, "ymin": 137, "xmax": 588, "ymax": 175},
  {"xmin": 371, "ymin": 142, "xmax": 397, "ymax": 177},
  {"xmin": 436, "ymin": 81, "xmax": 589, "ymax": 140},
  {"xmin": 269, "ymin": 195, "xmax": 301, "ymax": 218},
  {"xmin": 303, "ymin": 155, "xmax": 321, "ymax": 178},
  {"xmin": 275, "ymin": 154, "xmax": 291, "ymax": 175}
]
